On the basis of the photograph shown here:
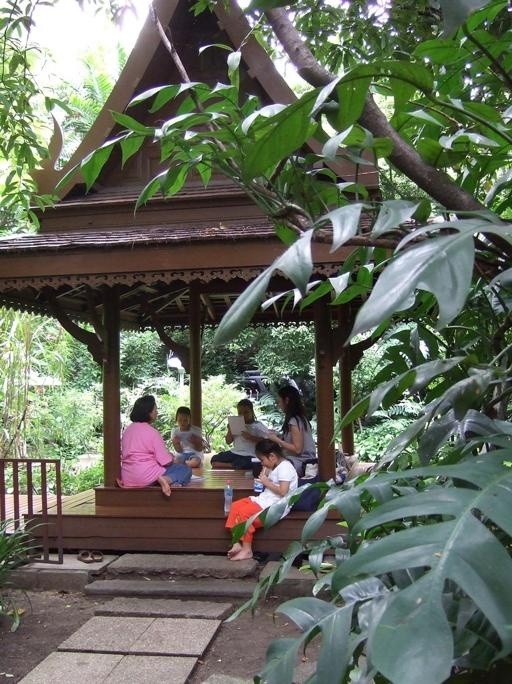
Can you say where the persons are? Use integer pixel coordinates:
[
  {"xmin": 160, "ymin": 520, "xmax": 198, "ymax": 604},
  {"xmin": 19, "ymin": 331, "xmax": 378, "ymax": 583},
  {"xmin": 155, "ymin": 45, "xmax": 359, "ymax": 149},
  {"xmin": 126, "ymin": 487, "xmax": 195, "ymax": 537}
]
[
  {"xmin": 252, "ymin": 386, "xmax": 316, "ymax": 479},
  {"xmin": 171, "ymin": 407, "xmax": 202, "ymax": 467},
  {"xmin": 121, "ymin": 396, "xmax": 192, "ymax": 496},
  {"xmin": 210, "ymin": 399, "xmax": 268, "ymax": 470},
  {"xmin": 225, "ymin": 439, "xmax": 298, "ymax": 560}
]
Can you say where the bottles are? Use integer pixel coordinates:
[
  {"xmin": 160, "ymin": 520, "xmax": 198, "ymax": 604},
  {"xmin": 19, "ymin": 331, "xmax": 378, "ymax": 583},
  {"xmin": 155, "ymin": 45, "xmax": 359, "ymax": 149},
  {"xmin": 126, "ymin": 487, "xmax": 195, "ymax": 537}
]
[
  {"xmin": 252, "ymin": 465, "xmax": 265, "ymax": 497},
  {"xmin": 223, "ymin": 481, "xmax": 233, "ymax": 513}
]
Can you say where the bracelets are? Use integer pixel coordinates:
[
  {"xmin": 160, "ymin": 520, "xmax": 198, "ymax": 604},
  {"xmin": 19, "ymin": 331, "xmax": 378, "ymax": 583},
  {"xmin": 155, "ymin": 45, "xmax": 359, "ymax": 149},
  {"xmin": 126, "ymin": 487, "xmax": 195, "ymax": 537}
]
[{"xmin": 279, "ymin": 440, "xmax": 284, "ymax": 448}]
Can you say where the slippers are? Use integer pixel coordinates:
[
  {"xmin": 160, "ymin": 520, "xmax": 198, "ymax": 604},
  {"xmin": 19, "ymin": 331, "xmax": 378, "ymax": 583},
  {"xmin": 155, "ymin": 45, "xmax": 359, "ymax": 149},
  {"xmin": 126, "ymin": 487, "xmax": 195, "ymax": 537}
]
[{"xmin": 77, "ymin": 551, "xmax": 103, "ymax": 563}]
